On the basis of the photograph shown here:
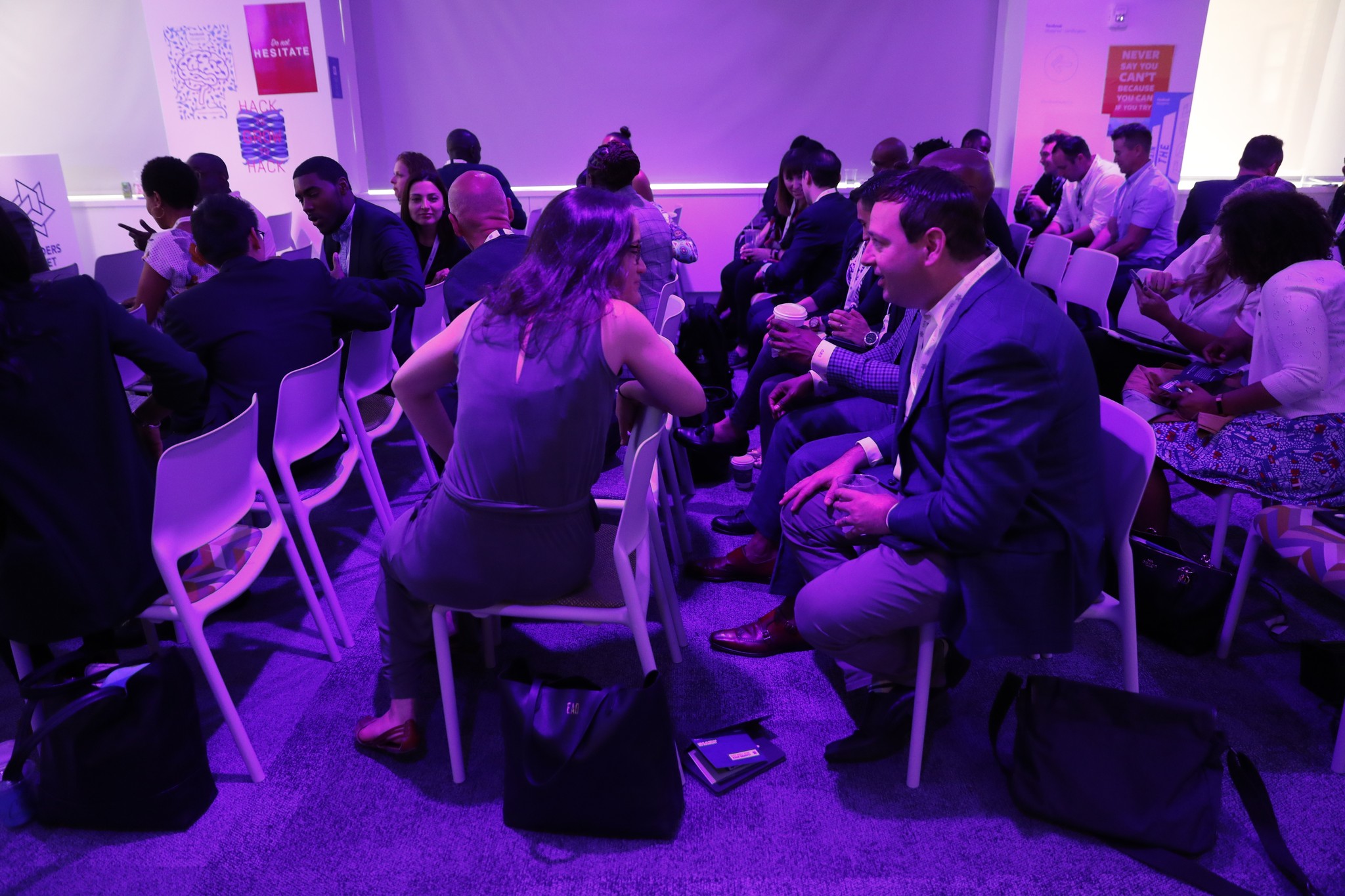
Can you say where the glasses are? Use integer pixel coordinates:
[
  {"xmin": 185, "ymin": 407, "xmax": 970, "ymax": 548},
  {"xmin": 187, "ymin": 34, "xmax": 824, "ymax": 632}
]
[
  {"xmin": 870, "ymin": 160, "xmax": 878, "ymax": 168},
  {"xmin": 629, "ymin": 239, "xmax": 643, "ymax": 264},
  {"xmin": 254, "ymin": 225, "xmax": 265, "ymax": 242}
]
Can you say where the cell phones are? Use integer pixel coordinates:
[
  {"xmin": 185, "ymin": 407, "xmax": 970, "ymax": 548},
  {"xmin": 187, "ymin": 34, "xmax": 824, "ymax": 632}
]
[{"xmin": 1129, "ymin": 269, "xmax": 1143, "ymax": 290}]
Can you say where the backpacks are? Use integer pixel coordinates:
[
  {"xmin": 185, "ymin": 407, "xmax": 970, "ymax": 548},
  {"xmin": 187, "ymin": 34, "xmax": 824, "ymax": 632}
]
[{"xmin": 678, "ymin": 295, "xmax": 733, "ymax": 405}]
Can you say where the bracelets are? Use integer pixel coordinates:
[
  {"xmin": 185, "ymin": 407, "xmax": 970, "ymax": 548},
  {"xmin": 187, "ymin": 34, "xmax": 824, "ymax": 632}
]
[
  {"xmin": 1215, "ymin": 393, "xmax": 1224, "ymax": 416},
  {"xmin": 617, "ymin": 383, "xmax": 635, "ymax": 402},
  {"xmin": 770, "ymin": 248, "xmax": 776, "ymax": 259},
  {"xmin": 775, "ymin": 248, "xmax": 781, "ymax": 260}
]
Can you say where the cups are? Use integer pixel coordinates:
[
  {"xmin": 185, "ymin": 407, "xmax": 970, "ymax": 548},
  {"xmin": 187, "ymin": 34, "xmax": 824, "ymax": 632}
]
[
  {"xmin": 836, "ymin": 474, "xmax": 879, "ymax": 536},
  {"xmin": 743, "ymin": 229, "xmax": 762, "ymax": 260},
  {"xmin": 731, "ymin": 454, "xmax": 755, "ymax": 489},
  {"xmin": 773, "ymin": 303, "xmax": 808, "ymax": 352}
]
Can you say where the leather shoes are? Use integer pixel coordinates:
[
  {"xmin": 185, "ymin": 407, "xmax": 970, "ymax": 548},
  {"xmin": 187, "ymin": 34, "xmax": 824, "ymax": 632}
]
[
  {"xmin": 711, "ymin": 598, "xmax": 815, "ymax": 657},
  {"xmin": 687, "ymin": 545, "xmax": 775, "ymax": 583}
]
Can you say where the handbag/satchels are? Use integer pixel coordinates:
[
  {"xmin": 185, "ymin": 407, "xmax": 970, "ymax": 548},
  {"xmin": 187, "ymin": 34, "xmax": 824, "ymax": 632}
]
[
  {"xmin": 4, "ymin": 641, "xmax": 217, "ymax": 835},
  {"xmin": 988, "ymin": 672, "xmax": 1315, "ymax": 896},
  {"xmin": 1127, "ymin": 527, "xmax": 1233, "ymax": 656},
  {"xmin": 677, "ymin": 382, "xmax": 732, "ymax": 484},
  {"xmin": 497, "ymin": 665, "xmax": 684, "ymax": 840}
]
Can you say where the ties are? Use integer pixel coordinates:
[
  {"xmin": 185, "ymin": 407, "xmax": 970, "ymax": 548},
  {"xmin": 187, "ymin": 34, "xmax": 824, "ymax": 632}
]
[
  {"xmin": 1075, "ymin": 183, "xmax": 1083, "ymax": 211},
  {"xmin": 891, "ymin": 312, "xmax": 921, "ymax": 463}
]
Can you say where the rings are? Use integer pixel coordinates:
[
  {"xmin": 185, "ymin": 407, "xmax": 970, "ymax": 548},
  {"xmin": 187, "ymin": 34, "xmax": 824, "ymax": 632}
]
[{"xmin": 839, "ymin": 323, "xmax": 844, "ymax": 331}]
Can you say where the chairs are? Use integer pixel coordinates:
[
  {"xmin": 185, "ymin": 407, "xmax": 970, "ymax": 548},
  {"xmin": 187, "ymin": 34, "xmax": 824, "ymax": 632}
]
[
  {"xmin": 651, "ymin": 273, "xmax": 700, "ymax": 498},
  {"xmin": 658, "ymin": 294, "xmax": 695, "ymax": 571},
  {"xmin": 342, "ymin": 303, "xmax": 440, "ymax": 524},
  {"xmin": 409, "ymin": 278, "xmax": 450, "ymax": 355},
  {"xmin": 1214, "ymin": 503, "xmax": 1345, "ymax": 777},
  {"xmin": 999, "ymin": 222, "xmax": 1192, "ymax": 352},
  {"xmin": 251, "ymin": 335, "xmax": 389, "ymax": 652},
  {"xmin": 670, "ymin": 206, "xmax": 682, "ymax": 226},
  {"xmin": 898, "ymin": 392, "xmax": 1164, "ymax": 787},
  {"xmin": 428, "ymin": 409, "xmax": 688, "ymax": 792},
  {"xmin": 524, "ymin": 207, "xmax": 542, "ymax": 234},
  {"xmin": 589, "ymin": 335, "xmax": 687, "ymax": 663},
  {"xmin": 131, "ymin": 389, "xmax": 344, "ymax": 787}
]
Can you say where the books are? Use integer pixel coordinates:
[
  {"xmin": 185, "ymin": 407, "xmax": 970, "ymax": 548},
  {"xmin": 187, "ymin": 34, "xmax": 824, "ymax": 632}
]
[
  {"xmin": 682, "ymin": 714, "xmax": 788, "ymax": 796},
  {"xmin": 1159, "ymin": 360, "xmax": 1233, "ymax": 395}
]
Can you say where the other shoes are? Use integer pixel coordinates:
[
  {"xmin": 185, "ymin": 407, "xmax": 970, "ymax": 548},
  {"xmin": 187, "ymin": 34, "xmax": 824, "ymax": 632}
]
[
  {"xmin": 711, "ymin": 508, "xmax": 754, "ymax": 535},
  {"xmin": 726, "ymin": 349, "xmax": 749, "ymax": 369},
  {"xmin": 352, "ymin": 717, "xmax": 423, "ymax": 762},
  {"xmin": 673, "ymin": 424, "xmax": 748, "ymax": 457},
  {"xmin": 825, "ymin": 637, "xmax": 971, "ymax": 765}
]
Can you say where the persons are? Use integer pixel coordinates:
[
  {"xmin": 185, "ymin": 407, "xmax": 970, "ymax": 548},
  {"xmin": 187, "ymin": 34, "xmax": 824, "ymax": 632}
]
[
  {"xmin": 354, "ymin": 183, "xmax": 708, "ymax": 762},
  {"xmin": 1013, "ymin": 122, "xmax": 1299, "ymax": 404},
  {"xmin": 1127, "ymin": 190, "xmax": 1345, "ymax": 556},
  {"xmin": 0, "ymin": 196, "xmax": 211, "ymax": 729},
  {"xmin": 772, "ymin": 164, "xmax": 1111, "ymax": 764},
  {"xmin": 87, "ymin": 130, "xmax": 699, "ymax": 656},
  {"xmin": 673, "ymin": 126, "xmax": 1013, "ymax": 661}
]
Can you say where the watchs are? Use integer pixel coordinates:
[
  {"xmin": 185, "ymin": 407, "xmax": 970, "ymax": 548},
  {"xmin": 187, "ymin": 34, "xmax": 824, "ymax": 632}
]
[
  {"xmin": 808, "ymin": 315, "xmax": 820, "ymax": 331},
  {"xmin": 864, "ymin": 330, "xmax": 880, "ymax": 350}
]
